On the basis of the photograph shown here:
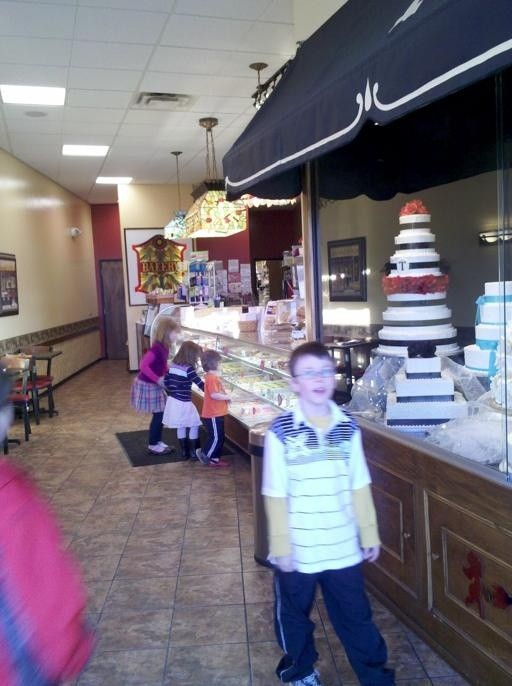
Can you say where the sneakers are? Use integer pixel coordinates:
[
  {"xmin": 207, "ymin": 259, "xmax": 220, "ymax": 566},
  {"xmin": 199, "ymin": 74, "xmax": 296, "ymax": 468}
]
[
  {"xmin": 208, "ymin": 458, "xmax": 231, "ymax": 467},
  {"xmin": 195, "ymin": 448, "xmax": 209, "ymax": 465},
  {"xmin": 287, "ymin": 667, "xmax": 323, "ymax": 686}
]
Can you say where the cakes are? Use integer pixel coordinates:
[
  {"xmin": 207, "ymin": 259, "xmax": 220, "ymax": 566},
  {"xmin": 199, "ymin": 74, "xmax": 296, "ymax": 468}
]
[
  {"xmin": 377, "ymin": 200, "xmax": 460, "ymax": 355},
  {"xmin": 490, "ymin": 321, "xmax": 512, "ymax": 411},
  {"xmin": 463, "ymin": 280, "xmax": 512, "ymax": 376},
  {"xmin": 386, "ymin": 341, "xmax": 468, "ymax": 431}
]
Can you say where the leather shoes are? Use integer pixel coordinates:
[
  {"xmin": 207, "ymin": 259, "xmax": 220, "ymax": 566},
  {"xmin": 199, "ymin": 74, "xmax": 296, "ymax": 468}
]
[{"xmin": 145, "ymin": 441, "xmax": 176, "ymax": 456}]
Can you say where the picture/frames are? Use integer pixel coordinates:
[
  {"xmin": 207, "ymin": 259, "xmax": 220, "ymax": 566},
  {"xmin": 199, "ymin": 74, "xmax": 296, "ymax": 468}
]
[
  {"xmin": 1, "ymin": 251, "xmax": 21, "ymax": 318},
  {"xmin": 326, "ymin": 235, "xmax": 368, "ymax": 302}
]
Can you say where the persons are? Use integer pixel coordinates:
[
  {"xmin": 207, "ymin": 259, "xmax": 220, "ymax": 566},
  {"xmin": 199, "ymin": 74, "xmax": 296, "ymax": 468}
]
[
  {"xmin": 259, "ymin": 340, "xmax": 398, "ymax": 686},
  {"xmin": 0, "ymin": 370, "xmax": 96, "ymax": 686},
  {"xmin": 194, "ymin": 349, "xmax": 232, "ymax": 467},
  {"xmin": 128, "ymin": 317, "xmax": 181, "ymax": 456},
  {"xmin": 161, "ymin": 340, "xmax": 204, "ymax": 459}
]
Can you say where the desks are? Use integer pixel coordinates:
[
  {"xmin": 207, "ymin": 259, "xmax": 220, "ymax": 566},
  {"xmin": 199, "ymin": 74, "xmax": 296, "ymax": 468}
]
[{"xmin": 323, "ymin": 335, "xmax": 378, "ymax": 405}]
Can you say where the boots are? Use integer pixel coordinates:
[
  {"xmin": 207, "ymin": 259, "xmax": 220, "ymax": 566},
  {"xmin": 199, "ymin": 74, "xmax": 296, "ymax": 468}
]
[
  {"xmin": 188, "ymin": 437, "xmax": 200, "ymax": 461},
  {"xmin": 179, "ymin": 438, "xmax": 189, "ymax": 459}
]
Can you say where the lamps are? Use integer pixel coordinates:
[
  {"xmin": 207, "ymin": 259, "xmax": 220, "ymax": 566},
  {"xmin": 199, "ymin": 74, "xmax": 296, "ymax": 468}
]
[
  {"xmin": 242, "ymin": 62, "xmax": 299, "ymax": 213},
  {"xmin": 183, "ymin": 114, "xmax": 247, "ymax": 240},
  {"xmin": 163, "ymin": 149, "xmax": 197, "ymax": 240},
  {"xmin": 477, "ymin": 225, "xmax": 511, "ymax": 248}
]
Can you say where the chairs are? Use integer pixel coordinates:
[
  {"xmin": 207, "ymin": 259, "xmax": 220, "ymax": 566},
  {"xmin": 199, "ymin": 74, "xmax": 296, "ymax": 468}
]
[
  {"xmin": 1, "ymin": 344, "xmax": 66, "ymax": 457},
  {"xmin": 321, "ymin": 335, "xmax": 364, "ymax": 379}
]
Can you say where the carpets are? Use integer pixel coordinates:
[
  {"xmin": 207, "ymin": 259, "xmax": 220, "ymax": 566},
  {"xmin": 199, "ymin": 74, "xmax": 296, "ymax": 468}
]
[{"xmin": 111, "ymin": 425, "xmax": 239, "ymax": 471}]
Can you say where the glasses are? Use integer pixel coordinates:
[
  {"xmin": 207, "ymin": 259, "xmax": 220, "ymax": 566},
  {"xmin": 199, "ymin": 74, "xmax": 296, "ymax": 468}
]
[{"xmin": 293, "ymin": 366, "xmax": 337, "ymax": 380}]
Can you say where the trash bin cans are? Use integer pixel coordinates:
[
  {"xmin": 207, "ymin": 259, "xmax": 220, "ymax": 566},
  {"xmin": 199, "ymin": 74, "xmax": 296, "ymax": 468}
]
[{"xmin": 248, "ymin": 420, "xmax": 275, "ymax": 568}]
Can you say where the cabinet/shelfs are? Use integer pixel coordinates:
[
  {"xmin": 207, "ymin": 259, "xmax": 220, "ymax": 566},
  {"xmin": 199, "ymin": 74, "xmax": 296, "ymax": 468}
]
[
  {"xmin": 159, "ymin": 307, "xmax": 325, "ymax": 462},
  {"xmin": 342, "ymin": 408, "xmax": 511, "ymax": 685}
]
